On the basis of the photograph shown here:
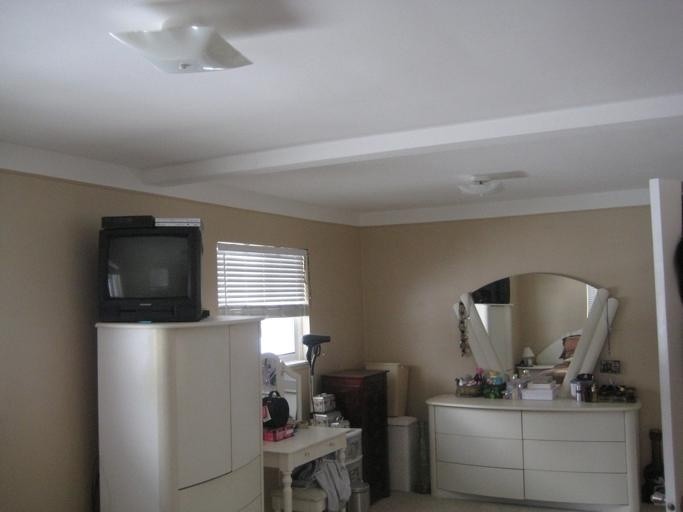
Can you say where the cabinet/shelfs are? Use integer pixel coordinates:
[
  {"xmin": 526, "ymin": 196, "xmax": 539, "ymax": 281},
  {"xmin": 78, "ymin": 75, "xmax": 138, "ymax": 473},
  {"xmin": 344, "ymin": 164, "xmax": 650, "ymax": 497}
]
[
  {"xmin": 91, "ymin": 313, "xmax": 268, "ymax": 512},
  {"xmin": 316, "ymin": 363, "xmax": 394, "ymax": 501},
  {"xmin": 423, "ymin": 393, "xmax": 647, "ymax": 512}
]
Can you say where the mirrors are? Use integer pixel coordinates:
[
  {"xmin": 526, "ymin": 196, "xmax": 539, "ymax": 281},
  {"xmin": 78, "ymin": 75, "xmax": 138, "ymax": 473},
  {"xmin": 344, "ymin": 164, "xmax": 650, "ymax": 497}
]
[{"xmin": 451, "ymin": 269, "xmax": 620, "ymax": 400}]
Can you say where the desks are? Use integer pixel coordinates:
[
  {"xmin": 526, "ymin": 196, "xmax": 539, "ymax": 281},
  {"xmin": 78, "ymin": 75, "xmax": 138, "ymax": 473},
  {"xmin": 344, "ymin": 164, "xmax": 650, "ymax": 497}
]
[{"xmin": 258, "ymin": 422, "xmax": 353, "ymax": 512}]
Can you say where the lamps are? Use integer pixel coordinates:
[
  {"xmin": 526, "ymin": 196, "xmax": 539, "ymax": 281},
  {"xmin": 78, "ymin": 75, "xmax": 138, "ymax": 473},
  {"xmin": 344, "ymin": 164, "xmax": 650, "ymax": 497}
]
[
  {"xmin": 519, "ymin": 346, "xmax": 537, "ymax": 367},
  {"xmin": 454, "ymin": 173, "xmax": 503, "ymax": 199},
  {"xmin": 106, "ymin": 14, "xmax": 254, "ymax": 77}
]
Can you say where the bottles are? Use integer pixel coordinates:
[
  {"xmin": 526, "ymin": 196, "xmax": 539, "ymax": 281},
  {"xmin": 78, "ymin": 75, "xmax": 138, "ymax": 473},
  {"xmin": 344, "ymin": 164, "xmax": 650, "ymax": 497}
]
[
  {"xmin": 574, "ymin": 385, "xmax": 583, "ymax": 402},
  {"xmin": 588, "ymin": 382, "xmax": 600, "ymax": 402}
]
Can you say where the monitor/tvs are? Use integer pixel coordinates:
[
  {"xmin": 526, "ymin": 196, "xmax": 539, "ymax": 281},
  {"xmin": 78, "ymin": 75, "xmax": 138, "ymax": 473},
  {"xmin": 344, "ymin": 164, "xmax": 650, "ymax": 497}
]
[
  {"xmin": 471, "ymin": 277, "xmax": 510, "ymax": 304},
  {"xmin": 99, "ymin": 227, "xmax": 201, "ymax": 322}
]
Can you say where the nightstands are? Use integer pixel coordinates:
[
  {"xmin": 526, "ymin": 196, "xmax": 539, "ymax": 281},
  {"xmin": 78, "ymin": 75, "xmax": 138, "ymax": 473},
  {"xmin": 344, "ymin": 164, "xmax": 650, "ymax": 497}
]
[{"xmin": 513, "ymin": 364, "xmax": 552, "ymax": 382}]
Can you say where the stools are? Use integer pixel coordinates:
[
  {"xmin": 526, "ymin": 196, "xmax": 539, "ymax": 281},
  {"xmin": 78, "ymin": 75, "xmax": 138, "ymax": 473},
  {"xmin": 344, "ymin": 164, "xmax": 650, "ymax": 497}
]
[{"xmin": 270, "ymin": 478, "xmax": 331, "ymax": 511}]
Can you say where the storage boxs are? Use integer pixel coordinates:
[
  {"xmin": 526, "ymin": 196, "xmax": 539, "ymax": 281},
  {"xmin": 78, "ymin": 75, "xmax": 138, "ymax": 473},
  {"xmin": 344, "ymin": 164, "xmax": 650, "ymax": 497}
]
[
  {"xmin": 344, "ymin": 457, "xmax": 363, "ymax": 486},
  {"xmin": 319, "ymin": 427, "xmax": 362, "ymax": 465}
]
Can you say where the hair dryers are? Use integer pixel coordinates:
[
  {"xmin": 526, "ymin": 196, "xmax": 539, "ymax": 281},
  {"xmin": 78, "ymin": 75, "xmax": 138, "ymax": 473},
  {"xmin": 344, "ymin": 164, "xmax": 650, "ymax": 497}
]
[{"xmin": 302, "ymin": 333, "xmax": 331, "ymax": 369}]
